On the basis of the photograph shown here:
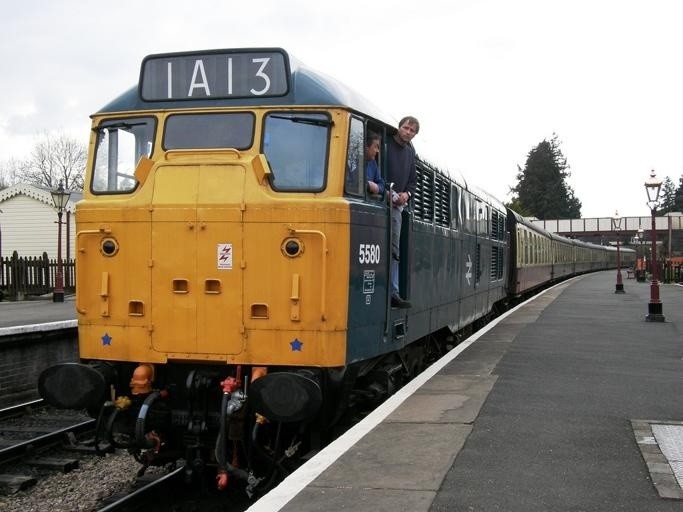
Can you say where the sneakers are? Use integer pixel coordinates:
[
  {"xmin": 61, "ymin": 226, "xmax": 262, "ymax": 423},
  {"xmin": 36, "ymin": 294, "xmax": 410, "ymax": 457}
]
[{"xmin": 392, "ymin": 293, "xmax": 411, "ymax": 309}]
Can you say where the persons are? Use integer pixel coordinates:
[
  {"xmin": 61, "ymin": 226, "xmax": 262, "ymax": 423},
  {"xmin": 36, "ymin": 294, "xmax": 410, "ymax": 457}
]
[
  {"xmin": 386, "ymin": 115, "xmax": 420, "ymax": 309},
  {"xmin": 367, "ymin": 129, "xmax": 386, "ymax": 195}
]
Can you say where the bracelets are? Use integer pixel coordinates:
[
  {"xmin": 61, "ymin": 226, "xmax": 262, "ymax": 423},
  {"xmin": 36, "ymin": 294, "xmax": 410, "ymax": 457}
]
[{"xmin": 407, "ymin": 191, "xmax": 411, "ymax": 197}]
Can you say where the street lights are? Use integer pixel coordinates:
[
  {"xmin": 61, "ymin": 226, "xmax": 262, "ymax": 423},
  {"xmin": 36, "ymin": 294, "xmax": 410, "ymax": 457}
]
[
  {"xmin": 610, "ymin": 210, "xmax": 624, "ymax": 293},
  {"xmin": 632, "ymin": 233, "xmax": 639, "ymax": 268},
  {"xmin": 642, "ymin": 168, "xmax": 664, "ymax": 321},
  {"xmin": 50, "ymin": 175, "xmax": 71, "ymax": 302},
  {"xmin": 637, "ymin": 224, "xmax": 646, "ymax": 281}
]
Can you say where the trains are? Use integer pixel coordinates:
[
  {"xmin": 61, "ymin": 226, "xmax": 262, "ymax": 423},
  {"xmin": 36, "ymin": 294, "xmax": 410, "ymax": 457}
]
[{"xmin": 37, "ymin": 42, "xmax": 648, "ymax": 502}]
